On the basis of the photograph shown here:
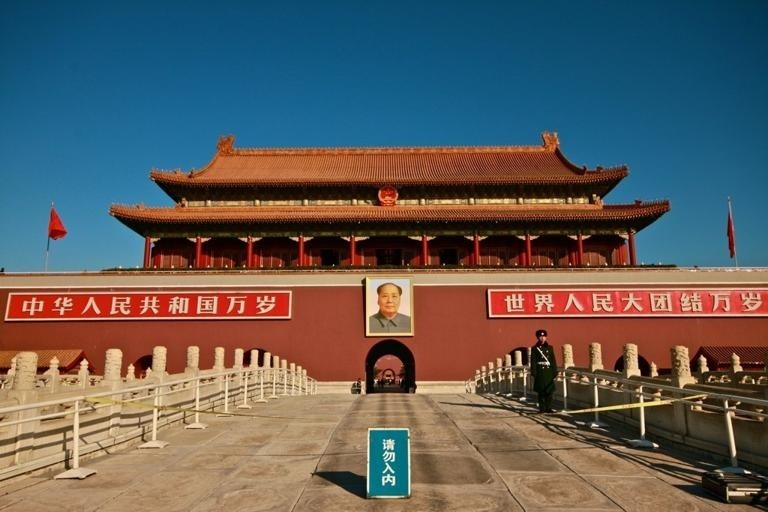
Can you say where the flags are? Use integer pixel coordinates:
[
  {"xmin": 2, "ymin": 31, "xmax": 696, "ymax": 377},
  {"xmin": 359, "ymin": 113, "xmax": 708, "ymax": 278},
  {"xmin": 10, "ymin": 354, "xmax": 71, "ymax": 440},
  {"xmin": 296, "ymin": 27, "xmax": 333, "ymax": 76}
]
[
  {"xmin": 725, "ymin": 204, "xmax": 736, "ymax": 259},
  {"xmin": 47, "ymin": 204, "xmax": 68, "ymax": 242}
]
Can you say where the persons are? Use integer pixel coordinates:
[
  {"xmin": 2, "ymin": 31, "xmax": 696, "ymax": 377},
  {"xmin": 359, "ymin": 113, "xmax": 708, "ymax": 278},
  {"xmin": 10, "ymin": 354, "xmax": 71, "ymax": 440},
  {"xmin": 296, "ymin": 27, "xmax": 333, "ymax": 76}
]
[
  {"xmin": 369, "ymin": 283, "xmax": 411, "ymax": 333},
  {"xmin": 355, "ymin": 378, "xmax": 362, "ymax": 393},
  {"xmin": 377, "ymin": 377, "xmax": 417, "ymax": 393},
  {"xmin": 531, "ymin": 329, "xmax": 557, "ymax": 413}
]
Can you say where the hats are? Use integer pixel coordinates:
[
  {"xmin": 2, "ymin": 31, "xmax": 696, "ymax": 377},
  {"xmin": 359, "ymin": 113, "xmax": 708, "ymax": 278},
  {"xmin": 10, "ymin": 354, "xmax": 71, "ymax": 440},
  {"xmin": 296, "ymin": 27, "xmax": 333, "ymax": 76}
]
[{"xmin": 535, "ymin": 330, "xmax": 547, "ymax": 336}]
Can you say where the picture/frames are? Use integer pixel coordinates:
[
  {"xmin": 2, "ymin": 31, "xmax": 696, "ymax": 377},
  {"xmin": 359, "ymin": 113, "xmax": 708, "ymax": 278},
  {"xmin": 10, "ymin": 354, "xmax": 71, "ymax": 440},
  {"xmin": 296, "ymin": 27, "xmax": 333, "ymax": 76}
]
[{"xmin": 364, "ymin": 273, "xmax": 416, "ymax": 338}]
